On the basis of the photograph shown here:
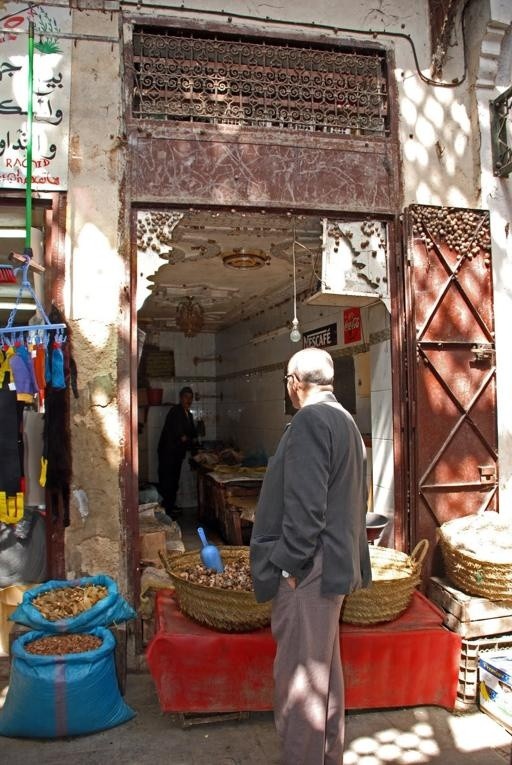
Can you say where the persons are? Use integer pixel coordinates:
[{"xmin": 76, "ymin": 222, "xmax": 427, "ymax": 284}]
[
  {"xmin": 157, "ymin": 386, "xmax": 200, "ymax": 519},
  {"xmin": 248, "ymin": 346, "xmax": 373, "ymax": 765}
]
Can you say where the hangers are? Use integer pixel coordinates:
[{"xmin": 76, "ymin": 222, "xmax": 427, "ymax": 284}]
[{"xmin": 0, "ymin": 256, "xmax": 67, "ymax": 348}]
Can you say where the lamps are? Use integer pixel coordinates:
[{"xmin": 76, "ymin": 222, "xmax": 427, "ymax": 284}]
[
  {"xmin": 176, "ymin": 295, "xmax": 204, "ymax": 337},
  {"xmin": 222, "ymin": 248, "xmax": 266, "ymax": 269},
  {"xmin": 289, "ymin": 240, "xmax": 321, "ymax": 343}
]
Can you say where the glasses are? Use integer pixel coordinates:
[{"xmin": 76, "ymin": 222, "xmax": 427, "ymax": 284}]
[{"xmin": 284, "ymin": 375, "xmax": 291, "ymax": 379}]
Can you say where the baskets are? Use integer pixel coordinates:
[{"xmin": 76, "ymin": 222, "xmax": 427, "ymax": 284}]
[
  {"xmin": 434, "ymin": 526, "xmax": 512, "ymax": 602},
  {"xmin": 340, "ymin": 538, "xmax": 429, "ymax": 627},
  {"xmin": 157, "ymin": 546, "xmax": 273, "ymax": 633}
]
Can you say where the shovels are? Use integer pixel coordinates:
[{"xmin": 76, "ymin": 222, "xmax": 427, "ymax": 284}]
[{"xmin": 196, "ymin": 527, "xmax": 224, "ymax": 573}]
